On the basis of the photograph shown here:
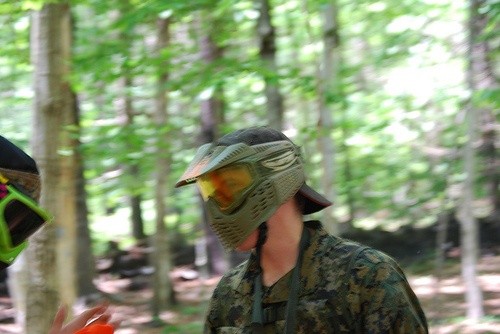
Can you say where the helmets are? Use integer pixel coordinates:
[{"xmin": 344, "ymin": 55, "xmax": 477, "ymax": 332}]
[
  {"xmin": 0, "ymin": 136, "xmax": 42, "ymax": 274},
  {"xmin": 173, "ymin": 125, "xmax": 307, "ymax": 253}
]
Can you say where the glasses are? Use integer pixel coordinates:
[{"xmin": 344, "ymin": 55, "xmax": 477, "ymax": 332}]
[
  {"xmin": 196, "ymin": 145, "xmax": 257, "ymax": 218},
  {"xmin": 0, "ymin": 176, "xmax": 51, "ymax": 267}
]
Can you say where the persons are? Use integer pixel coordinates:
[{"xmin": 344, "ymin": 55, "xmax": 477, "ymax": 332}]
[
  {"xmin": 172, "ymin": 124, "xmax": 431, "ymax": 334},
  {"xmin": 0, "ymin": 135, "xmax": 122, "ymax": 334}
]
